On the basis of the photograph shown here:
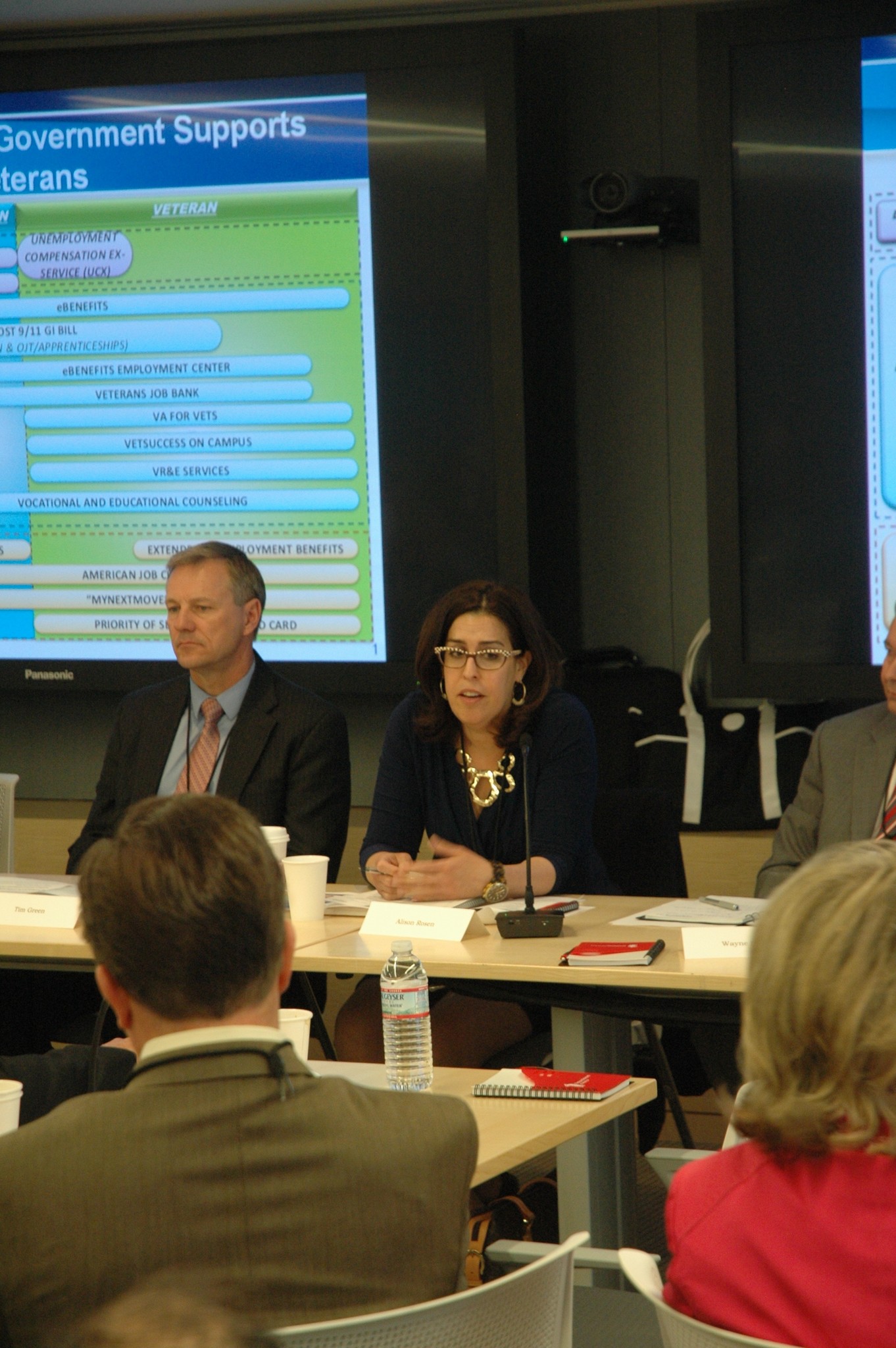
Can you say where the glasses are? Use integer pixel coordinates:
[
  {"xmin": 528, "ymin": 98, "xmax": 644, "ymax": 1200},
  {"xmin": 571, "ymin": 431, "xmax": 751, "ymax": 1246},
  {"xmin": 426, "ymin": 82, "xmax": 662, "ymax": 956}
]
[{"xmin": 434, "ymin": 646, "xmax": 524, "ymax": 671}]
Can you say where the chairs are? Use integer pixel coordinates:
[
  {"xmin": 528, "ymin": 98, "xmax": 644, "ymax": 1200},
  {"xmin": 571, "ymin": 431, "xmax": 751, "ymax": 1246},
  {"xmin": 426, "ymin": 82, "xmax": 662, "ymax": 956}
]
[{"xmin": 265, "ymin": 1229, "xmax": 807, "ymax": 1348}]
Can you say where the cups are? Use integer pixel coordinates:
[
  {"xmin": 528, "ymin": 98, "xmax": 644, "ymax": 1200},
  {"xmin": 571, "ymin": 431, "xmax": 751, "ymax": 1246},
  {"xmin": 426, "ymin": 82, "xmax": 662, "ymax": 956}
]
[
  {"xmin": 260, "ymin": 825, "xmax": 291, "ymax": 868},
  {"xmin": 0, "ymin": 1079, "xmax": 23, "ymax": 1097},
  {"xmin": 0, "ymin": 1091, "xmax": 23, "ymax": 1138},
  {"xmin": 281, "ymin": 854, "xmax": 329, "ymax": 932},
  {"xmin": 278, "ymin": 1008, "xmax": 314, "ymax": 1065}
]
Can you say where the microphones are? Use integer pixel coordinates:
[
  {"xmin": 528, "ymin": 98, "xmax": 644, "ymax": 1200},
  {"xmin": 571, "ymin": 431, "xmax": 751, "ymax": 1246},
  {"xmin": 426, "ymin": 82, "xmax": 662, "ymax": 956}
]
[{"xmin": 494, "ymin": 733, "xmax": 564, "ymax": 939}]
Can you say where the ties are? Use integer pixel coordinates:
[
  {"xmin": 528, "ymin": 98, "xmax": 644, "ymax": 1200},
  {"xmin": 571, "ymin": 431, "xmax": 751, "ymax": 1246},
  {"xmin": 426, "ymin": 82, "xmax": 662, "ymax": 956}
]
[
  {"xmin": 875, "ymin": 762, "xmax": 896, "ymax": 844},
  {"xmin": 176, "ymin": 698, "xmax": 225, "ymax": 798}
]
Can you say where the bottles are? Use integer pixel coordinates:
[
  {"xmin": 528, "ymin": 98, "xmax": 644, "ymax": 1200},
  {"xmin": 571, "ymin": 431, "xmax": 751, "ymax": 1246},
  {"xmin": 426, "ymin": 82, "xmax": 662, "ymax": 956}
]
[{"xmin": 379, "ymin": 939, "xmax": 435, "ymax": 1089}]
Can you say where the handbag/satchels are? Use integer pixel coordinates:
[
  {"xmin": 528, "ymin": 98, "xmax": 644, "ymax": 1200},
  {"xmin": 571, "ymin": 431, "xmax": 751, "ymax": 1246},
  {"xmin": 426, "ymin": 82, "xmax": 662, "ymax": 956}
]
[{"xmin": 462, "ymin": 1168, "xmax": 562, "ymax": 1291}]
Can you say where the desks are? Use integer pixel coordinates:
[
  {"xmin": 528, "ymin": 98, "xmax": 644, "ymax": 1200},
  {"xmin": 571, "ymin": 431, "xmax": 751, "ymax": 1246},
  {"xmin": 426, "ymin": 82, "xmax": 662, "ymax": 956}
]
[{"xmin": 0, "ymin": 871, "xmax": 775, "ymax": 1187}]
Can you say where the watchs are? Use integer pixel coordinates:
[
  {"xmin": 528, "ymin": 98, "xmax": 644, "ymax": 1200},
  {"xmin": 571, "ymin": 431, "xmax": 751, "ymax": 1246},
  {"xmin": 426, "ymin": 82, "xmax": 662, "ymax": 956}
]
[{"xmin": 482, "ymin": 858, "xmax": 509, "ymax": 904}]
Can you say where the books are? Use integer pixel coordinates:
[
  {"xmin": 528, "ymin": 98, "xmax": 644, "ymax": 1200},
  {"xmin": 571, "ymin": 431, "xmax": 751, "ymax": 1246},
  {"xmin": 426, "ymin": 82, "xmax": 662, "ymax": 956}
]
[
  {"xmin": 636, "ymin": 893, "xmax": 772, "ymax": 926},
  {"xmin": 323, "ymin": 885, "xmax": 578, "ymax": 917},
  {"xmin": 471, "ymin": 1068, "xmax": 632, "ymax": 1101},
  {"xmin": 559, "ymin": 939, "xmax": 665, "ymax": 966}
]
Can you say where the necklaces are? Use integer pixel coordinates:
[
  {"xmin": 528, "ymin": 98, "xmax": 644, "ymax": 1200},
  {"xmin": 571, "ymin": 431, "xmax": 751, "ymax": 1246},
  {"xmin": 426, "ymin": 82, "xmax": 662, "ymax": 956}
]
[{"xmin": 453, "ymin": 747, "xmax": 516, "ymax": 807}]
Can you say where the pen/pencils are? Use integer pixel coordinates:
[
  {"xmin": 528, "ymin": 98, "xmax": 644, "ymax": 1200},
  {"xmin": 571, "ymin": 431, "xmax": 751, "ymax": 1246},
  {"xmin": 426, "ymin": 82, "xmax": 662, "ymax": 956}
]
[
  {"xmin": 560, "ymin": 952, "xmax": 572, "ymax": 961},
  {"xmin": 358, "ymin": 866, "xmax": 381, "ymax": 875},
  {"xmin": 699, "ymin": 896, "xmax": 739, "ymax": 911}
]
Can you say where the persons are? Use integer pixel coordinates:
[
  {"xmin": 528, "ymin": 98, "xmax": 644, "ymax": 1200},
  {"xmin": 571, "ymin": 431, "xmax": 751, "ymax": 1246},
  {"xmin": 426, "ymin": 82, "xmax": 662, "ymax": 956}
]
[
  {"xmin": 333, "ymin": 579, "xmax": 599, "ymax": 1068},
  {"xmin": 1, "ymin": 791, "xmax": 479, "ymax": 1348},
  {"xmin": 754, "ymin": 603, "xmax": 896, "ymax": 899},
  {"xmin": 64, "ymin": 542, "xmax": 352, "ymax": 884},
  {"xmin": 659, "ymin": 840, "xmax": 896, "ymax": 1348}
]
